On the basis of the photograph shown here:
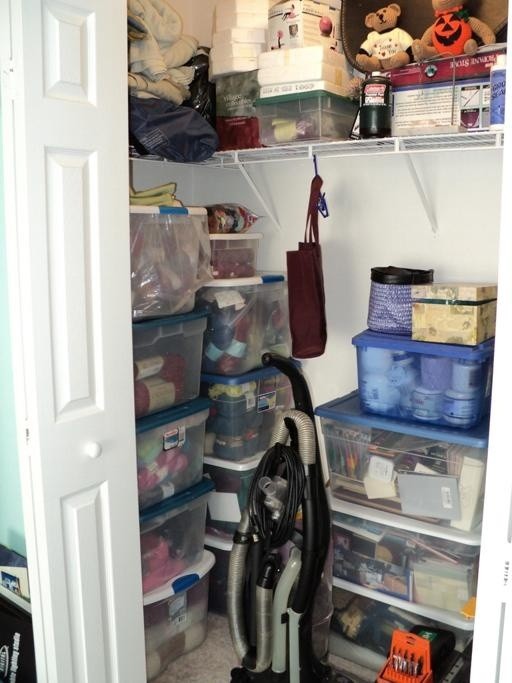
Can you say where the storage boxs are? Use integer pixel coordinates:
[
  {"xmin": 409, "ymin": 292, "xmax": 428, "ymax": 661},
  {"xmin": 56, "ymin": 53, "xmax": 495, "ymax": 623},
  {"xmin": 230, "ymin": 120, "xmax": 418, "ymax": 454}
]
[
  {"xmin": 352, "ymin": 329, "xmax": 495, "ymax": 431},
  {"xmin": 253, "ymin": 90, "xmax": 358, "ymax": 146},
  {"xmin": 411, "ymin": 281, "xmax": 498, "ymax": 346},
  {"xmin": 314, "ymin": 388, "xmax": 489, "ymax": 532},
  {"xmin": 329, "ymin": 577, "xmax": 474, "ymax": 673},
  {"xmin": 381, "ymin": 44, "xmax": 506, "ymax": 136},
  {"xmin": 332, "ymin": 496, "xmax": 480, "ymax": 632}
]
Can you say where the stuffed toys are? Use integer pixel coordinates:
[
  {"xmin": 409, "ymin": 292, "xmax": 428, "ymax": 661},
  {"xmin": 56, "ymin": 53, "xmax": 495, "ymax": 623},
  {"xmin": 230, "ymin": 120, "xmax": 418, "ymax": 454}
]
[
  {"xmin": 412, "ymin": 0, "xmax": 496, "ymax": 60},
  {"xmin": 356, "ymin": 4, "xmax": 413, "ymax": 71}
]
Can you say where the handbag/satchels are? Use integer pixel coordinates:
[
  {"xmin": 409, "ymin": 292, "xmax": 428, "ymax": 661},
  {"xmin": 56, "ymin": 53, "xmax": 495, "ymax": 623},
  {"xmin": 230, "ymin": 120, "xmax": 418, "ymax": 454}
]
[{"xmin": 287, "ymin": 241, "xmax": 327, "ymax": 358}]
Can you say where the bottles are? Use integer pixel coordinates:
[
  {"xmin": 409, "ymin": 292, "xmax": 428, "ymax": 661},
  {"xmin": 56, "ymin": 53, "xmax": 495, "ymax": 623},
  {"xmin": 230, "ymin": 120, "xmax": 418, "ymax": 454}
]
[
  {"xmin": 349, "ymin": 108, "xmax": 361, "ymax": 140},
  {"xmin": 360, "ymin": 71, "xmax": 393, "ymax": 139},
  {"xmin": 488, "ymin": 53, "xmax": 506, "ymax": 131}
]
